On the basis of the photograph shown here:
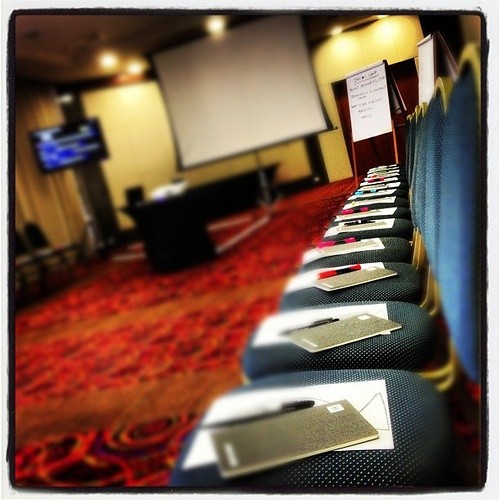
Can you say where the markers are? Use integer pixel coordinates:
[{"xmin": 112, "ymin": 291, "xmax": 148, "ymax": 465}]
[{"xmin": 318, "ymin": 163, "xmax": 398, "ymax": 278}]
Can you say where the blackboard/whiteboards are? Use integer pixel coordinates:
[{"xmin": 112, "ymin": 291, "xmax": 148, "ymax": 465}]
[
  {"xmin": 345, "ymin": 62, "xmax": 393, "ymax": 141},
  {"xmin": 416, "ymin": 33, "xmax": 456, "ymax": 108}
]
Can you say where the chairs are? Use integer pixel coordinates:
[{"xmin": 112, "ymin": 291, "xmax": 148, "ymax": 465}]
[
  {"xmin": 166, "ymin": 41, "xmax": 486, "ymax": 492},
  {"xmin": 23, "ymin": 218, "xmax": 90, "ymax": 280},
  {"xmin": 15, "ymin": 232, "xmax": 55, "ymax": 303}
]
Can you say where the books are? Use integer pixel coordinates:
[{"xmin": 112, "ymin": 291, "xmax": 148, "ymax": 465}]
[
  {"xmin": 288, "ymin": 313, "xmax": 402, "ymax": 353},
  {"xmin": 314, "ymin": 267, "xmax": 397, "ymax": 292},
  {"xmin": 209, "ymin": 400, "xmax": 378, "ymax": 478},
  {"xmin": 314, "ymin": 240, "xmax": 380, "ymax": 254},
  {"xmin": 336, "ymin": 209, "xmax": 382, "ymax": 220},
  {"xmin": 335, "ymin": 221, "xmax": 386, "ymax": 232}
]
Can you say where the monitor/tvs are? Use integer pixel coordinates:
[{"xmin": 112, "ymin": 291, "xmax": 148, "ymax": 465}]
[{"xmin": 28, "ymin": 118, "xmax": 110, "ymax": 175}]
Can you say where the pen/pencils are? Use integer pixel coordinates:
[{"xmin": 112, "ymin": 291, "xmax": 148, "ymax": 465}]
[
  {"xmin": 209, "ymin": 399, "xmax": 314, "ymax": 428},
  {"xmin": 280, "ymin": 316, "xmax": 338, "ymax": 334}
]
